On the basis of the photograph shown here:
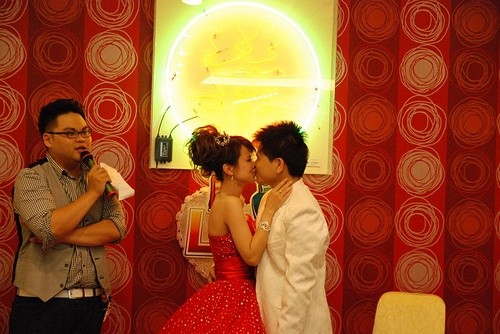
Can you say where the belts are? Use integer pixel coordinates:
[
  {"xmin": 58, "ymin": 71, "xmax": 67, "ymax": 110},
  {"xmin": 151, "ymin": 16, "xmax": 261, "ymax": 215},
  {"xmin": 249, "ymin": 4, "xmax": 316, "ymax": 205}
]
[{"xmin": 17, "ymin": 288, "xmax": 104, "ymax": 299}]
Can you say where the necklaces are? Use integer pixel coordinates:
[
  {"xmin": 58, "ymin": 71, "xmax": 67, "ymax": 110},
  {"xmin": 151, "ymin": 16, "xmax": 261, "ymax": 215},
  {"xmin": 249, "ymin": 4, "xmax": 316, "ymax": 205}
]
[{"xmin": 217, "ymin": 190, "xmax": 224, "ymax": 194}]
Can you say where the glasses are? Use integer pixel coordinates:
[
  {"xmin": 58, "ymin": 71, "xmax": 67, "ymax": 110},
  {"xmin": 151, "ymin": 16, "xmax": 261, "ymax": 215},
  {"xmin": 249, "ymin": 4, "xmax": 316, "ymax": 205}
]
[{"xmin": 48, "ymin": 129, "xmax": 92, "ymax": 139}]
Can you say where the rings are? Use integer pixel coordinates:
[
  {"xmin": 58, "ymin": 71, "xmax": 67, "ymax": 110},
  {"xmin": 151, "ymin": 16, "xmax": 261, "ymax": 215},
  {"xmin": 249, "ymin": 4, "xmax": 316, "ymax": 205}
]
[{"xmin": 279, "ymin": 190, "xmax": 282, "ymax": 193}]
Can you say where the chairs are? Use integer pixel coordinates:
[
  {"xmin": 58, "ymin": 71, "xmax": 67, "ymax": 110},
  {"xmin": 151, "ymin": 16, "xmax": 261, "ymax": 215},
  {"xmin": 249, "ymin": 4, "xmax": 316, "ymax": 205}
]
[{"xmin": 373, "ymin": 292, "xmax": 446, "ymax": 334}]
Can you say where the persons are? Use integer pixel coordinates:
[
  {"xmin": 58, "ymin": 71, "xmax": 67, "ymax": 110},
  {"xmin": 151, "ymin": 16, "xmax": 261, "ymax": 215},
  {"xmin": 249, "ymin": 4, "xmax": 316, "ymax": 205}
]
[
  {"xmin": 209, "ymin": 121, "xmax": 333, "ymax": 334},
  {"xmin": 158, "ymin": 125, "xmax": 293, "ymax": 334},
  {"xmin": 8, "ymin": 97, "xmax": 127, "ymax": 334}
]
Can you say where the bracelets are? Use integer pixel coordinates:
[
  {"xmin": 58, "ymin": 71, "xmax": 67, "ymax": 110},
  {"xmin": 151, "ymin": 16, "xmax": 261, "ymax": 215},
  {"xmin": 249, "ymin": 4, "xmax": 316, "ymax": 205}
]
[{"xmin": 257, "ymin": 221, "xmax": 271, "ymax": 232}]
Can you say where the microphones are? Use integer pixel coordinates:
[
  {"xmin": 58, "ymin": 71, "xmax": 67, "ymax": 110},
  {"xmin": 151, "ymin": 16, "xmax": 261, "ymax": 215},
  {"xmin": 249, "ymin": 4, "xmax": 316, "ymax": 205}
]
[{"xmin": 80, "ymin": 151, "xmax": 116, "ymax": 197}]
